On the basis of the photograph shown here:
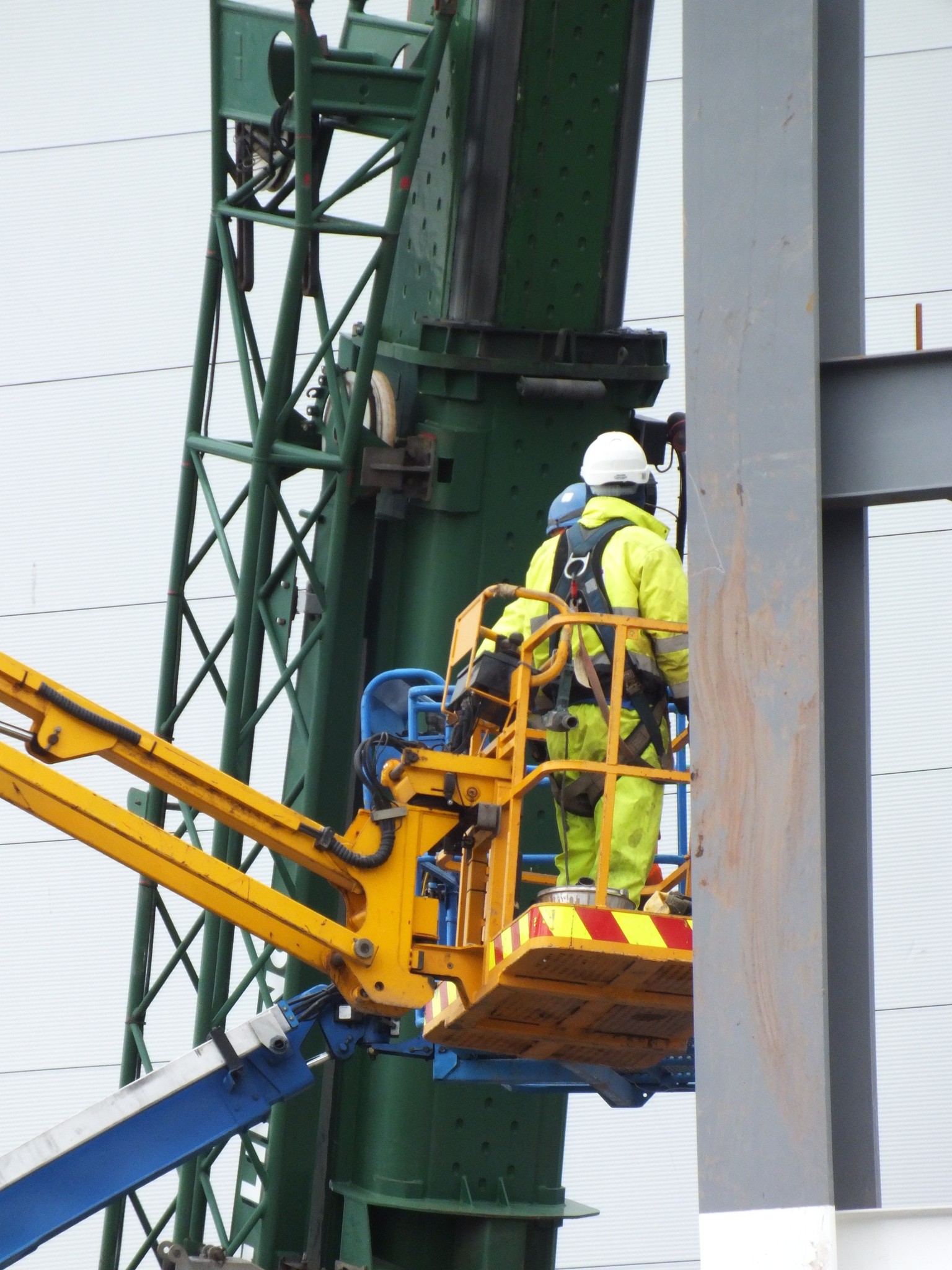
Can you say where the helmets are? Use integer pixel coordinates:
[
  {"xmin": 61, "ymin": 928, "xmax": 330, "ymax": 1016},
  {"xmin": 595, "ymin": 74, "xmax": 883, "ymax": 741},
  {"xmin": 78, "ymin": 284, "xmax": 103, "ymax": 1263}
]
[
  {"xmin": 544, "ymin": 478, "xmax": 586, "ymax": 531},
  {"xmin": 579, "ymin": 429, "xmax": 649, "ymax": 485}
]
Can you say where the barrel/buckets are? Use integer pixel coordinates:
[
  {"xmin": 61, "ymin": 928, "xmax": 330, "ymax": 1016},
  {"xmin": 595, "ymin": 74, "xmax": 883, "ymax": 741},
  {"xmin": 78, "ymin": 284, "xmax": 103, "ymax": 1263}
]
[{"xmin": 537, "ymin": 885, "xmax": 636, "ymax": 910}]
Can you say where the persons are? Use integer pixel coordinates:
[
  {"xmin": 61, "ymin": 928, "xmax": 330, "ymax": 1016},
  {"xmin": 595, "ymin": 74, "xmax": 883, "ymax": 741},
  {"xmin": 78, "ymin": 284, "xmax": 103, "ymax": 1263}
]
[{"xmin": 473, "ymin": 431, "xmax": 689, "ymax": 911}]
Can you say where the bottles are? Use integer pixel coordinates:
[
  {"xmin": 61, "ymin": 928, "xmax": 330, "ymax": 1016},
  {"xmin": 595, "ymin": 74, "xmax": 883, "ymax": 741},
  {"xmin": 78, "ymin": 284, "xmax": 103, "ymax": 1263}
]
[{"xmin": 576, "ymin": 877, "xmax": 596, "ymax": 886}]
